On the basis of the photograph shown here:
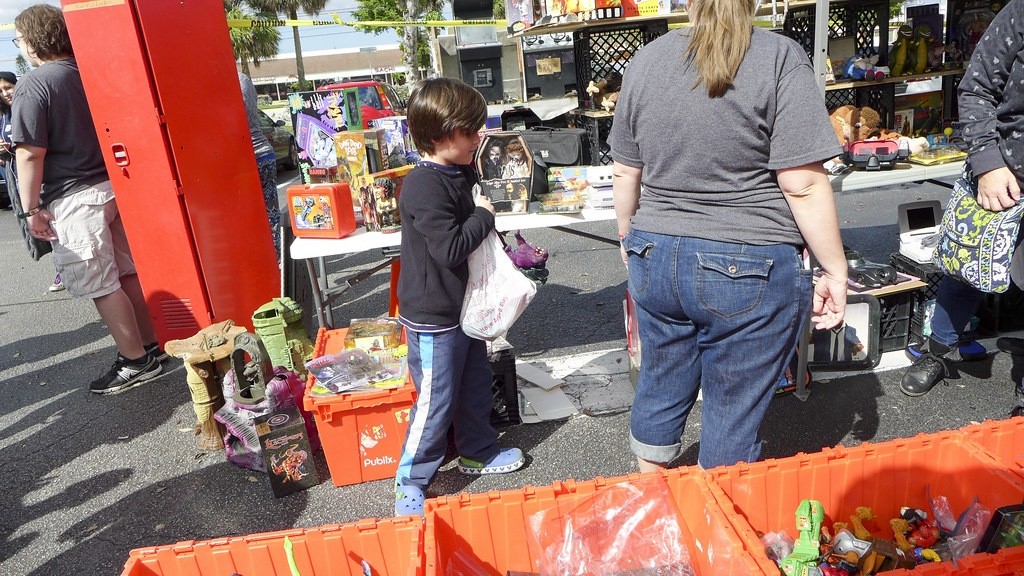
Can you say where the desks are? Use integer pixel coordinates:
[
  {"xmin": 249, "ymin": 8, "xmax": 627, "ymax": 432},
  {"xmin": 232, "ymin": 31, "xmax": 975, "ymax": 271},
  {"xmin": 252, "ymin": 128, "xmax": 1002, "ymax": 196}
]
[
  {"xmin": 826, "ymin": 68, "xmax": 966, "ymax": 119},
  {"xmin": 290, "ymin": 160, "xmax": 966, "ymax": 403}
]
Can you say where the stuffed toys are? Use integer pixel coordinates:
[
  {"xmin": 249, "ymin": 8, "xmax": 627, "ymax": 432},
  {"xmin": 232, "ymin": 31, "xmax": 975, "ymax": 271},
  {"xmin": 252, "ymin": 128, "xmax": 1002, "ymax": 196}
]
[
  {"xmin": 888, "ymin": 23, "xmax": 946, "ymax": 76},
  {"xmin": 846, "ymin": 56, "xmax": 885, "ymax": 81},
  {"xmin": 828, "ymin": 105, "xmax": 899, "ymax": 142},
  {"xmin": 587, "ymin": 74, "xmax": 619, "ymax": 112},
  {"xmin": 501, "ymin": 230, "xmax": 549, "ymax": 283}
]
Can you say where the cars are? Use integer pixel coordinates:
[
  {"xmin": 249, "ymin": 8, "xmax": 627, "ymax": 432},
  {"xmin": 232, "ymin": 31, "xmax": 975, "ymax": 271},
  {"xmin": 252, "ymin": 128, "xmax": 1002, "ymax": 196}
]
[{"xmin": 257, "ymin": 109, "xmax": 300, "ymax": 171}]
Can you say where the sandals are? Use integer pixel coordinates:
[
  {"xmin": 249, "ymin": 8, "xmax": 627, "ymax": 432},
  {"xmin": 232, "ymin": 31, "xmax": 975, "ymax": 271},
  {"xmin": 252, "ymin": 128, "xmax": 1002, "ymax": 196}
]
[
  {"xmin": 457, "ymin": 445, "xmax": 526, "ymax": 474},
  {"xmin": 394, "ymin": 479, "xmax": 425, "ymax": 518}
]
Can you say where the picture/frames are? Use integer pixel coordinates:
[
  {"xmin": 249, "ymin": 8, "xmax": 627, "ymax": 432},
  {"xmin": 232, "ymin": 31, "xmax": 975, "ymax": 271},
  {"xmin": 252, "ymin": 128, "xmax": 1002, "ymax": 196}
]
[{"xmin": 806, "ymin": 294, "xmax": 881, "ymax": 371}]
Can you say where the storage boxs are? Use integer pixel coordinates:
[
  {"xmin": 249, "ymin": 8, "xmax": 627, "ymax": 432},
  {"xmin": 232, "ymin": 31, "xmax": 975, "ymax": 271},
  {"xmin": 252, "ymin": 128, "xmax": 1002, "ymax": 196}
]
[
  {"xmin": 624, "ymin": 287, "xmax": 642, "ymax": 393},
  {"xmin": 118, "ymin": 511, "xmax": 427, "ymax": 576},
  {"xmin": 447, "ymin": 350, "xmax": 521, "ymax": 439},
  {"xmin": 287, "ymin": 86, "xmax": 364, "ymax": 185},
  {"xmin": 959, "ymin": 415, "xmax": 1024, "ymax": 482},
  {"xmin": 343, "ymin": 320, "xmax": 396, "ymax": 352},
  {"xmin": 368, "ymin": 116, "xmax": 421, "ymax": 168},
  {"xmin": 589, "ymin": 187, "xmax": 614, "ymax": 209},
  {"xmin": 878, "ymin": 291, "xmax": 912, "ymax": 352},
  {"xmin": 288, "ymin": 326, "xmax": 419, "ymax": 490},
  {"xmin": 356, "ymin": 164, "xmax": 416, "ymax": 234},
  {"xmin": 474, "ymin": 130, "xmax": 535, "ymax": 216},
  {"xmin": 420, "ymin": 462, "xmax": 767, "ymax": 576},
  {"xmin": 537, "ymin": 191, "xmax": 585, "ymax": 214},
  {"xmin": 998, "ymin": 277, "xmax": 1024, "ymax": 333},
  {"xmin": 889, "ymin": 249, "xmax": 1001, "ymax": 353},
  {"xmin": 309, "ymin": 163, "xmax": 339, "ymax": 184},
  {"xmin": 545, "ymin": 165, "xmax": 589, "ymax": 193},
  {"xmin": 286, "ymin": 180, "xmax": 359, "ymax": 240},
  {"xmin": 333, "ymin": 127, "xmax": 388, "ymax": 208},
  {"xmin": 254, "ymin": 404, "xmax": 321, "ymax": 498},
  {"xmin": 710, "ymin": 428, "xmax": 1024, "ymax": 576},
  {"xmin": 586, "ymin": 165, "xmax": 614, "ymax": 187}
]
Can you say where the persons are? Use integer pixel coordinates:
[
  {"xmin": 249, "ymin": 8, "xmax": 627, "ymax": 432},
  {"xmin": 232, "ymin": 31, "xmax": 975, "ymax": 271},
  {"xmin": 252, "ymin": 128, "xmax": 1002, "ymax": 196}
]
[
  {"xmin": 239, "ymin": 71, "xmax": 281, "ymax": 270},
  {"xmin": 394, "ymin": 76, "xmax": 525, "ymax": 517},
  {"xmin": 9, "ymin": 5, "xmax": 168, "ymax": 393},
  {"xmin": 484, "ymin": 139, "xmax": 529, "ymax": 213},
  {"xmin": 374, "ymin": 177, "xmax": 401, "ymax": 226},
  {"xmin": 0, "ymin": 71, "xmax": 65, "ymax": 291},
  {"xmin": 965, "ymin": 14, "xmax": 988, "ymax": 56},
  {"xmin": 946, "ymin": 41, "xmax": 964, "ymax": 63},
  {"xmin": 900, "ymin": 0, "xmax": 1024, "ymax": 401},
  {"xmin": 606, "ymin": 0, "xmax": 847, "ymax": 471}
]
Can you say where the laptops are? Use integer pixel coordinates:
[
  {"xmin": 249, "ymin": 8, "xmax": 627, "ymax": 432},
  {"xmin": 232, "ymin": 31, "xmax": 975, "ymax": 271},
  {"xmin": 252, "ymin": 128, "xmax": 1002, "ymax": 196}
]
[{"xmin": 898, "ymin": 200, "xmax": 945, "ymax": 263}]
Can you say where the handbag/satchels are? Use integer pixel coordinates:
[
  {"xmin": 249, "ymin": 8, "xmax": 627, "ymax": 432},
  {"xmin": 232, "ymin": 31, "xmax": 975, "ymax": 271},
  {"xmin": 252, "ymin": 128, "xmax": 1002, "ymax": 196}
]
[
  {"xmin": 3, "ymin": 153, "xmax": 52, "ymax": 261},
  {"xmin": 520, "ymin": 126, "xmax": 592, "ymax": 167},
  {"xmin": 920, "ymin": 176, "xmax": 1024, "ymax": 293},
  {"xmin": 459, "ymin": 226, "xmax": 537, "ymax": 341}
]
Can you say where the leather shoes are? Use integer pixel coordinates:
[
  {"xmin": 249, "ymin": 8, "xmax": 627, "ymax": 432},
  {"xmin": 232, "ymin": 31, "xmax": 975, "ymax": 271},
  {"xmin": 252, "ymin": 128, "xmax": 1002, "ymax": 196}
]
[{"xmin": 899, "ymin": 339, "xmax": 962, "ymax": 395}]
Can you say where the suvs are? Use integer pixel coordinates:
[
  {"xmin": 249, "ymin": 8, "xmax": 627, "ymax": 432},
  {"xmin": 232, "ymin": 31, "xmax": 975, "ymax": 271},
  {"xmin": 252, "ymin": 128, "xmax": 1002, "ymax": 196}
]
[{"xmin": 316, "ymin": 76, "xmax": 409, "ymax": 130}]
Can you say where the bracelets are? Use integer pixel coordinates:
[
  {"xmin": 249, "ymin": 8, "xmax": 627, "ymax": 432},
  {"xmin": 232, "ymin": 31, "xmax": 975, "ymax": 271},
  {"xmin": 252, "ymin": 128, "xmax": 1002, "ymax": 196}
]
[
  {"xmin": 18, "ymin": 205, "xmax": 43, "ymax": 219},
  {"xmin": 619, "ymin": 235, "xmax": 624, "ymax": 240}
]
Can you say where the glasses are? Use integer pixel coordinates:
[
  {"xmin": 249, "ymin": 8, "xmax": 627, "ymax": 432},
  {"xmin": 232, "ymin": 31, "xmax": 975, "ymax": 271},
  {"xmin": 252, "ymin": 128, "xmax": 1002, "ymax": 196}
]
[{"xmin": 13, "ymin": 36, "xmax": 25, "ymax": 48}]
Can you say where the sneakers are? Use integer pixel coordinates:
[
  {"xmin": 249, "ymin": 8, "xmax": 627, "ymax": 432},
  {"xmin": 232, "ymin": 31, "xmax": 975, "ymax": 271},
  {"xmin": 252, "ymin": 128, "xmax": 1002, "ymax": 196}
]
[
  {"xmin": 48, "ymin": 272, "xmax": 65, "ymax": 292},
  {"xmin": 89, "ymin": 352, "xmax": 163, "ymax": 393},
  {"xmin": 144, "ymin": 341, "xmax": 168, "ymax": 362}
]
